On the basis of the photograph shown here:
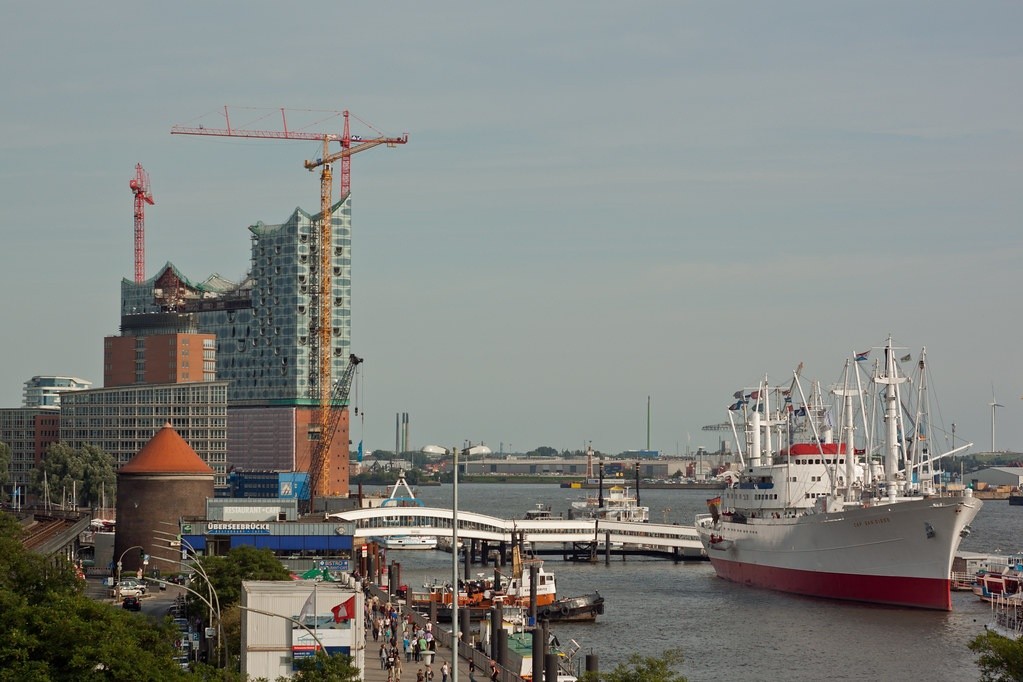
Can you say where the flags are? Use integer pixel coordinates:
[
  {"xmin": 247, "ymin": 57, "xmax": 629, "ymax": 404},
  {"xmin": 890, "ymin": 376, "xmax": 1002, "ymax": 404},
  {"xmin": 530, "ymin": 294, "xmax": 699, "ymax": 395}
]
[
  {"xmin": 331, "ymin": 595, "xmax": 355, "ymax": 623},
  {"xmin": 855, "ymin": 350, "xmax": 870, "ymax": 360},
  {"xmin": 298, "ymin": 590, "xmax": 316, "ymax": 624},
  {"xmin": 900, "ymin": 354, "xmax": 911, "ymax": 362},
  {"xmin": 782, "ymin": 389, "xmax": 794, "ymax": 411},
  {"xmin": 12, "ymin": 488, "xmax": 19, "ymax": 495},
  {"xmin": 707, "ymin": 496, "xmax": 720, "ymax": 506},
  {"xmin": 729, "ymin": 390, "xmax": 764, "ymax": 411}
]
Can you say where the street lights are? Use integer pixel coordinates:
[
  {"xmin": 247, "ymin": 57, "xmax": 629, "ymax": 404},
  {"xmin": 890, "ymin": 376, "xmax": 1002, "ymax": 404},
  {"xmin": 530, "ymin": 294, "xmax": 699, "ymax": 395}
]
[{"xmin": 450, "ymin": 447, "xmax": 494, "ymax": 682}]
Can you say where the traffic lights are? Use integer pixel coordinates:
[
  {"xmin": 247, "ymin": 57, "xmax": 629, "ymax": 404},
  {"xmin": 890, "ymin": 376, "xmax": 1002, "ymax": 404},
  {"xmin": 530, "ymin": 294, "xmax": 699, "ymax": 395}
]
[{"xmin": 141, "ymin": 550, "xmax": 145, "ymax": 559}]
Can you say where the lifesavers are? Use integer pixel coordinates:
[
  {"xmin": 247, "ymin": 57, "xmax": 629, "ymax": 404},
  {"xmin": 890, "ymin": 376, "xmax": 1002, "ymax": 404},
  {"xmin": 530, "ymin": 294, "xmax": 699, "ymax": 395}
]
[
  {"xmin": 561, "ymin": 607, "xmax": 569, "ymax": 616},
  {"xmin": 542, "ymin": 609, "xmax": 552, "ymax": 618},
  {"xmin": 504, "ymin": 598, "xmax": 511, "ymax": 605}
]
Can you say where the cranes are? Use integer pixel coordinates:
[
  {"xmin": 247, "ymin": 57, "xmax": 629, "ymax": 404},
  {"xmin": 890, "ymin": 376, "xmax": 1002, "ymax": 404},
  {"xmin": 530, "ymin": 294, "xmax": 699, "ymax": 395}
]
[{"xmin": 130, "ymin": 106, "xmax": 411, "ymax": 508}]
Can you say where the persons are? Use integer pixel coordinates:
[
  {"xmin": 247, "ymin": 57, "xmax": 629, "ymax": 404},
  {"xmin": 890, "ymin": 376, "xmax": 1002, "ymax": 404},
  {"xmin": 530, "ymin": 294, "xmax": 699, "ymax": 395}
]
[
  {"xmin": 353, "ymin": 570, "xmax": 452, "ymax": 682},
  {"xmin": 468, "ymin": 657, "xmax": 477, "ymax": 682},
  {"xmin": 490, "ymin": 661, "xmax": 498, "ymax": 682}
]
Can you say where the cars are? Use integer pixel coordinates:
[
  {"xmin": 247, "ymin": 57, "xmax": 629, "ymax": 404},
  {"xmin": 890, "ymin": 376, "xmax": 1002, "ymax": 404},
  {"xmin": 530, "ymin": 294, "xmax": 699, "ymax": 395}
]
[
  {"xmin": 169, "ymin": 575, "xmax": 185, "ymax": 586},
  {"xmin": 123, "ymin": 596, "xmax": 142, "ymax": 610},
  {"xmin": 110, "ymin": 575, "xmax": 147, "ymax": 599}
]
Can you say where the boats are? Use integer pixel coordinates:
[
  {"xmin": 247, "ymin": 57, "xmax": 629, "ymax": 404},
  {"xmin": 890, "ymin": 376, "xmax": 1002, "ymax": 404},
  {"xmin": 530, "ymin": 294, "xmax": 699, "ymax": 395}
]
[
  {"xmin": 692, "ymin": 327, "xmax": 983, "ymax": 614},
  {"xmin": 974, "ymin": 548, "xmax": 1023, "ymax": 608},
  {"xmin": 410, "ymin": 518, "xmax": 607, "ymax": 626},
  {"xmin": 526, "ymin": 501, "xmax": 555, "ymax": 521}
]
